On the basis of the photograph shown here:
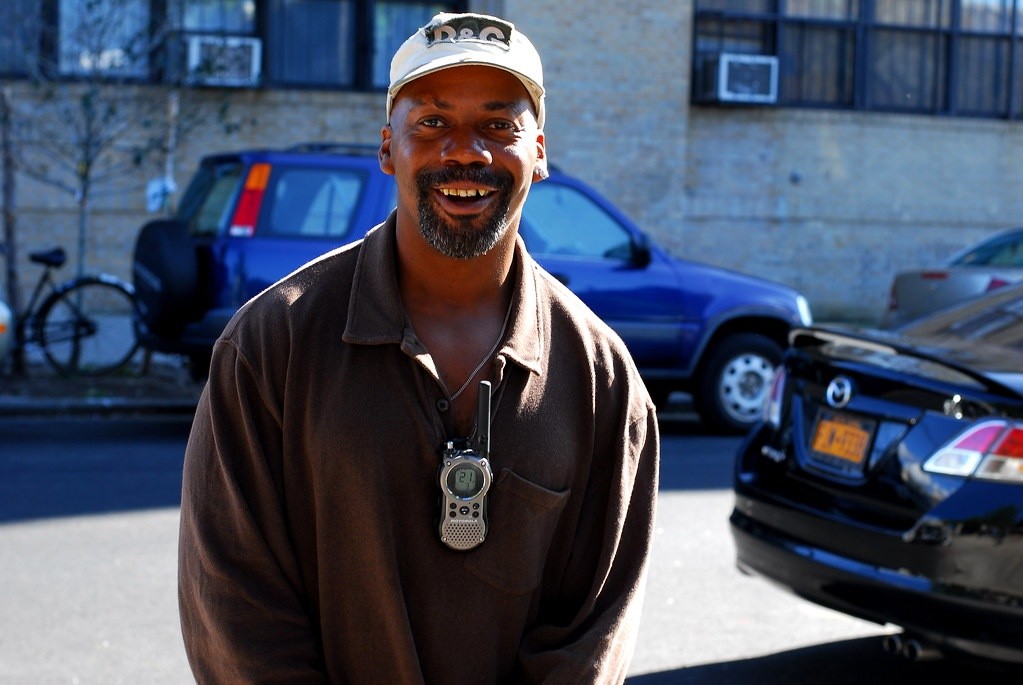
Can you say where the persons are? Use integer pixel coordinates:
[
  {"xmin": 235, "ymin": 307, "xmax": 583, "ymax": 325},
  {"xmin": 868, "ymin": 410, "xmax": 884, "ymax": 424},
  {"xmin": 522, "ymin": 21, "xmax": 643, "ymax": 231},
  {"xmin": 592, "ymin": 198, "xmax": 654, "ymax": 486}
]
[{"xmin": 177, "ymin": 13, "xmax": 660, "ymax": 685}]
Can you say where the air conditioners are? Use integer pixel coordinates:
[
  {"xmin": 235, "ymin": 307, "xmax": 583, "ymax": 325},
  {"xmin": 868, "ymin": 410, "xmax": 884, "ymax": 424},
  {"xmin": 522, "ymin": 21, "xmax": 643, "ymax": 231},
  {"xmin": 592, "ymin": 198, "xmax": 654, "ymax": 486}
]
[
  {"xmin": 697, "ymin": 52, "xmax": 780, "ymax": 105},
  {"xmin": 167, "ymin": 29, "xmax": 264, "ymax": 89}
]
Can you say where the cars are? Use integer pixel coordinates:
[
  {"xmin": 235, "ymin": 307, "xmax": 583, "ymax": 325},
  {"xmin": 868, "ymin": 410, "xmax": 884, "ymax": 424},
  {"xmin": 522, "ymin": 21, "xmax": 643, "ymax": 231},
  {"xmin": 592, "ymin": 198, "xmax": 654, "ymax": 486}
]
[
  {"xmin": 0, "ymin": 301, "xmax": 15, "ymax": 370},
  {"xmin": 879, "ymin": 225, "xmax": 1023, "ymax": 346},
  {"xmin": 727, "ymin": 277, "xmax": 1023, "ymax": 685}
]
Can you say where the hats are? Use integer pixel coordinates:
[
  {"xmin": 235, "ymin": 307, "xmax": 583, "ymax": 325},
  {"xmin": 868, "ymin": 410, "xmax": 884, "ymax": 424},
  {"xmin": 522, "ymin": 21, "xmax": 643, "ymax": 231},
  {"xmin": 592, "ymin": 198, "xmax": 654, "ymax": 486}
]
[{"xmin": 384, "ymin": 11, "xmax": 545, "ymax": 130}]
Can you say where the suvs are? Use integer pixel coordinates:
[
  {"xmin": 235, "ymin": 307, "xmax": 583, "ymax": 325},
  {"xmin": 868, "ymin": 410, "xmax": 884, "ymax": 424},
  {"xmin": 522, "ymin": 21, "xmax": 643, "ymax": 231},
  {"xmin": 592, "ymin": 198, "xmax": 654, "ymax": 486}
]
[{"xmin": 130, "ymin": 141, "xmax": 818, "ymax": 440}]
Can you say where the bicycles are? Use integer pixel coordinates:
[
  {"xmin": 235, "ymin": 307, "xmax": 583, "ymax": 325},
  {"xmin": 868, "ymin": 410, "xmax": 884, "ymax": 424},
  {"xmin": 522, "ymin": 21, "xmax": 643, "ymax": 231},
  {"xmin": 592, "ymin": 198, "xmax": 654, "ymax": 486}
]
[{"xmin": 0, "ymin": 240, "xmax": 144, "ymax": 380}]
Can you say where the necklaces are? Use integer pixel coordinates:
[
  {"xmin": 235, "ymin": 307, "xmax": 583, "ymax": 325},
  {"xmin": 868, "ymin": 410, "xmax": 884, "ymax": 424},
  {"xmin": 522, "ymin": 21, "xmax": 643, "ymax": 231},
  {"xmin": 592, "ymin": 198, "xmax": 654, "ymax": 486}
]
[{"xmin": 449, "ymin": 291, "xmax": 515, "ymax": 400}]
